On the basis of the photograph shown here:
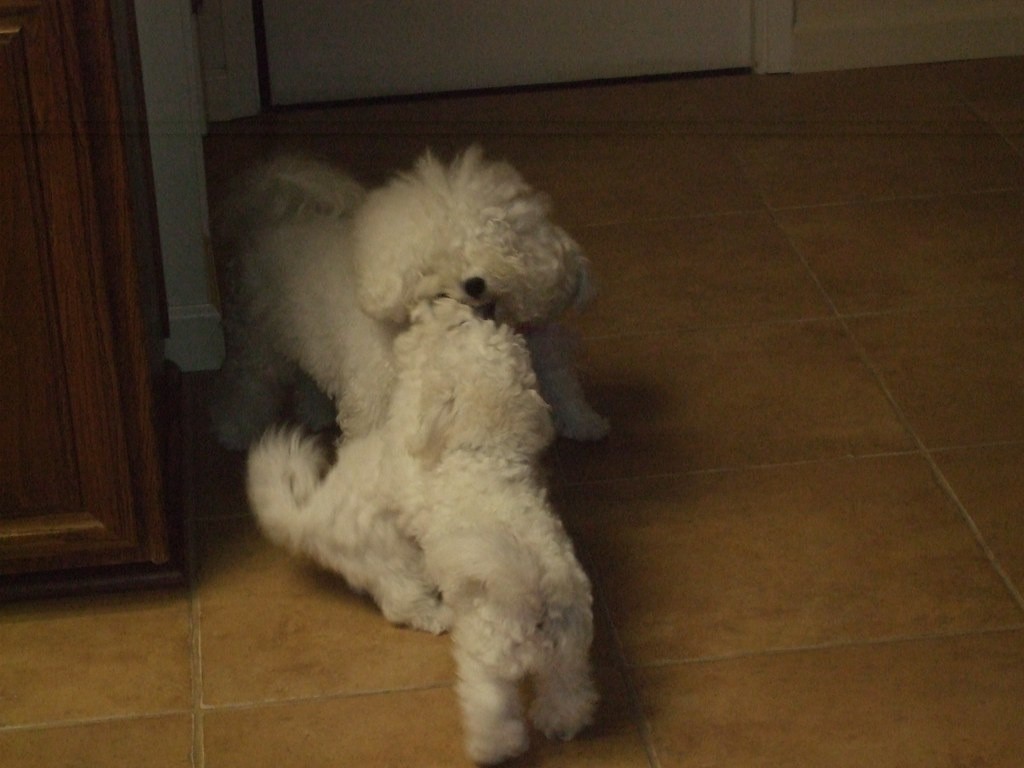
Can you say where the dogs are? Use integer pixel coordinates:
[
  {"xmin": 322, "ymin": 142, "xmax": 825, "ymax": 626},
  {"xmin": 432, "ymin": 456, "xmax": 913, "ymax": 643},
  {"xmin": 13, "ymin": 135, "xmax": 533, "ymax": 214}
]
[
  {"xmin": 375, "ymin": 293, "xmax": 601, "ymax": 767},
  {"xmin": 219, "ymin": 144, "xmax": 609, "ymax": 449},
  {"xmin": 243, "ymin": 424, "xmax": 452, "ymax": 635}
]
